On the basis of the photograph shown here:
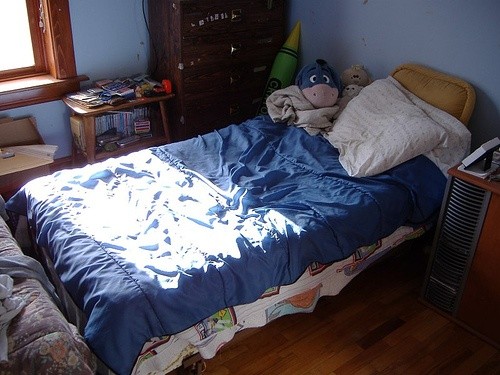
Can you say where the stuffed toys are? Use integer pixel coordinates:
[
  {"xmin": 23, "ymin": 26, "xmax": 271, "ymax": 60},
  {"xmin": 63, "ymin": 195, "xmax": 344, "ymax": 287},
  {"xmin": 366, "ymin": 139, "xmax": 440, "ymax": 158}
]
[
  {"xmin": 295, "ymin": 58, "xmax": 339, "ymax": 108},
  {"xmin": 340, "ymin": 64, "xmax": 373, "ymax": 87},
  {"xmin": 334, "ymin": 84, "xmax": 363, "ymax": 119}
]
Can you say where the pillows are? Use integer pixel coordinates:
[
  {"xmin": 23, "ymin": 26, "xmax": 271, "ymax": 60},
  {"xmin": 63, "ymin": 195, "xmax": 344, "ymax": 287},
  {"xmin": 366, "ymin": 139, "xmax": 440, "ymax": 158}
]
[
  {"xmin": 389, "ymin": 62, "xmax": 477, "ymax": 126},
  {"xmin": 384, "ymin": 78, "xmax": 470, "ymax": 173},
  {"xmin": 322, "ymin": 78, "xmax": 447, "ymax": 179}
]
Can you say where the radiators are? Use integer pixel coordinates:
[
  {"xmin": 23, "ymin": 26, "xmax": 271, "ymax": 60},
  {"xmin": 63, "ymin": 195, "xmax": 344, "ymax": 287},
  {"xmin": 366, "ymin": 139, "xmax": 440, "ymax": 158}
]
[{"xmin": 420, "ymin": 174, "xmax": 492, "ymax": 316}]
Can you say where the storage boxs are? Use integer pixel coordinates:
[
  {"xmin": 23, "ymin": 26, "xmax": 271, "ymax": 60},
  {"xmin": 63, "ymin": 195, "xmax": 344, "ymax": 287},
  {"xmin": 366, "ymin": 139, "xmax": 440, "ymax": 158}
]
[{"xmin": 0, "ymin": 116, "xmax": 56, "ymax": 200}]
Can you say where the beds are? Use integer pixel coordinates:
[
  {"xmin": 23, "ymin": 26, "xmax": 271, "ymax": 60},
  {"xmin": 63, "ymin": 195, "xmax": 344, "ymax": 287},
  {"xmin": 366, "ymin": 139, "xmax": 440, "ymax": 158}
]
[{"xmin": 4, "ymin": 86, "xmax": 461, "ymax": 374}]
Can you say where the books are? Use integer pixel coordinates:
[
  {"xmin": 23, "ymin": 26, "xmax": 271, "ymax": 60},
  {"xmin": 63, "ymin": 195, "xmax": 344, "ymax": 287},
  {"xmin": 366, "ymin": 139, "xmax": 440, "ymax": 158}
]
[
  {"xmin": 73, "ymin": 79, "xmax": 164, "ymax": 98},
  {"xmin": 69, "ymin": 108, "xmax": 153, "ymax": 150}
]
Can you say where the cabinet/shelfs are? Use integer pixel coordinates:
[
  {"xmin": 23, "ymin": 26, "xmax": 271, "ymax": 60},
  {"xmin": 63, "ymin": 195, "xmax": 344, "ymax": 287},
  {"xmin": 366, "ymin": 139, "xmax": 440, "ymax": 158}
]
[
  {"xmin": 63, "ymin": 81, "xmax": 178, "ymax": 168},
  {"xmin": 149, "ymin": 0, "xmax": 288, "ymax": 140}
]
